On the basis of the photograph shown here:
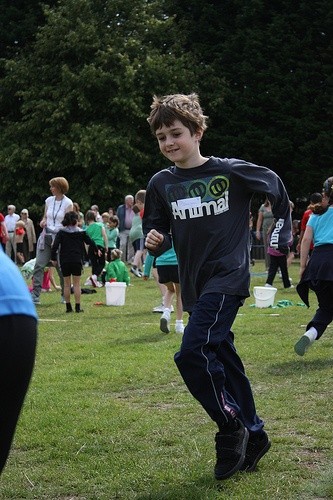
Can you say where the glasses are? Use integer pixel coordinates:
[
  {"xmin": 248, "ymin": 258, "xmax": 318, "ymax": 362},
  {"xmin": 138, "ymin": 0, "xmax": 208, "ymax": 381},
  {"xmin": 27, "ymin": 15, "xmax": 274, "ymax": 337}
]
[{"xmin": 22, "ymin": 213, "xmax": 27, "ymax": 215}]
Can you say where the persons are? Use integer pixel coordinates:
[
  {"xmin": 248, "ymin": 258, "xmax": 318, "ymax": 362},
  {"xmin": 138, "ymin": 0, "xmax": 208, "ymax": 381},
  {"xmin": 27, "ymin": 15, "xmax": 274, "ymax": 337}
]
[
  {"xmin": 142, "ymin": 96, "xmax": 294, "ymax": 480},
  {"xmin": 294, "ymin": 177, "xmax": 333, "ymax": 357},
  {"xmin": 0, "ymin": 188, "xmax": 160, "ymax": 291},
  {"xmin": 265, "ymin": 200, "xmax": 295, "ymax": 290},
  {"xmin": 255, "ymin": 188, "xmax": 293, "ymax": 281},
  {"xmin": 154, "ymin": 243, "xmax": 186, "ymax": 335},
  {"xmin": 29, "ymin": 177, "xmax": 74, "ymax": 305},
  {"xmin": 297, "ymin": 191, "xmax": 324, "ymax": 265},
  {"xmin": 0, "ymin": 244, "xmax": 38, "ymax": 475},
  {"xmin": 48, "ymin": 211, "xmax": 102, "ymax": 313}
]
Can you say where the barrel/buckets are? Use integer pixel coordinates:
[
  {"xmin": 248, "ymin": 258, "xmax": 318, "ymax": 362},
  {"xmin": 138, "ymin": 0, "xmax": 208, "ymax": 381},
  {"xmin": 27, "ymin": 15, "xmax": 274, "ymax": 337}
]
[
  {"xmin": 253, "ymin": 285, "xmax": 277, "ymax": 308},
  {"xmin": 104, "ymin": 282, "xmax": 127, "ymax": 306}
]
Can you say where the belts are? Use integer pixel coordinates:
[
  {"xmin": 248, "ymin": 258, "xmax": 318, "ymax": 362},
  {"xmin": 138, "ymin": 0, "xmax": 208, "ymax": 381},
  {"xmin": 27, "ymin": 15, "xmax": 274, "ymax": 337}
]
[{"xmin": 8, "ymin": 231, "xmax": 15, "ymax": 233}]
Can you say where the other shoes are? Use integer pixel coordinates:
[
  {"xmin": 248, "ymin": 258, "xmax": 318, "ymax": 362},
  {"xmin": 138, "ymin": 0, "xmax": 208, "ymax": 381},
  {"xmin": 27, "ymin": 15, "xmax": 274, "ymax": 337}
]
[
  {"xmin": 60, "ymin": 297, "xmax": 65, "ymax": 303},
  {"xmin": 285, "ymin": 285, "xmax": 296, "ymax": 289},
  {"xmin": 66, "ymin": 309, "xmax": 73, "ymax": 312},
  {"xmin": 32, "ymin": 298, "xmax": 40, "ymax": 304},
  {"xmin": 264, "ymin": 282, "xmax": 272, "ymax": 287},
  {"xmin": 152, "ymin": 304, "xmax": 174, "ymax": 312},
  {"xmin": 76, "ymin": 309, "xmax": 84, "ymax": 313},
  {"xmin": 131, "ymin": 268, "xmax": 143, "ymax": 277},
  {"xmin": 278, "ymin": 274, "xmax": 294, "ymax": 281}
]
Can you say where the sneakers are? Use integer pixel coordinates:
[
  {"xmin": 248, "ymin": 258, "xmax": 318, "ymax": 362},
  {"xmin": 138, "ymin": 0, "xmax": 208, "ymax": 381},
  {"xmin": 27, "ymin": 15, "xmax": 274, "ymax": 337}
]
[
  {"xmin": 242, "ymin": 431, "xmax": 271, "ymax": 472},
  {"xmin": 293, "ymin": 328, "xmax": 314, "ymax": 356},
  {"xmin": 160, "ymin": 313, "xmax": 170, "ymax": 333},
  {"xmin": 175, "ymin": 326, "xmax": 185, "ymax": 335},
  {"xmin": 90, "ymin": 275, "xmax": 97, "ymax": 286},
  {"xmin": 214, "ymin": 419, "xmax": 249, "ymax": 478}
]
